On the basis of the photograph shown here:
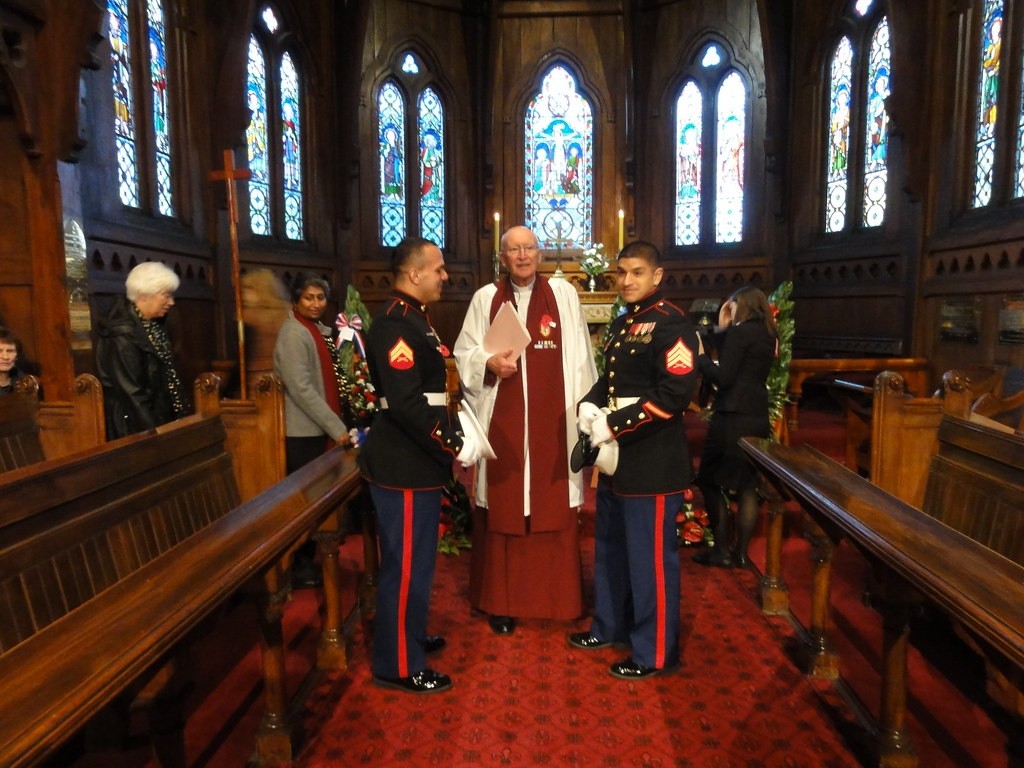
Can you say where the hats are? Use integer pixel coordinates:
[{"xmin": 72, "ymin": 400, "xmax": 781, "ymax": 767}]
[{"xmin": 570, "ymin": 434, "xmax": 600, "ymax": 472}]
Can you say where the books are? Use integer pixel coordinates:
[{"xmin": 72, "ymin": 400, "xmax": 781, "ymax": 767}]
[{"xmin": 483, "ymin": 301, "xmax": 531, "ymax": 380}]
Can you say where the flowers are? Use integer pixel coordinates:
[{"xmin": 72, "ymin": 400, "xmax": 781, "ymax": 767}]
[
  {"xmin": 676, "ymin": 490, "xmax": 714, "ymax": 548},
  {"xmin": 349, "ymin": 360, "xmax": 377, "ymax": 423},
  {"xmin": 435, "ymin": 494, "xmax": 471, "ymax": 555},
  {"xmin": 573, "ymin": 243, "xmax": 611, "ymax": 279}
]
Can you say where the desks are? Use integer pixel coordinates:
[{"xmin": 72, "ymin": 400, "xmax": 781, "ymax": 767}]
[
  {"xmin": 578, "ymin": 290, "xmax": 618, "ymax": 324},
  {"xmin": 738, "ymin": 434, "xmax": 1024, "ymax": 768},
  {"xmin": 0, "ymin": 438, "xmax": 379, "ymax": 768}
]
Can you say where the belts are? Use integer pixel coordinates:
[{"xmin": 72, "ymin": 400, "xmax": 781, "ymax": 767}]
[
  {"xmin": 380, "ymin": 392, "xmax": 450, "ymax": 409},
  {"xmin": 607, "ymin": 397, "xmax": 641, "ymax": 411}
]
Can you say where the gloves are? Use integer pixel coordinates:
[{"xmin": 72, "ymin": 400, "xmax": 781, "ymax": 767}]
[
  {"xmin": 578, "ymin": 402, "xmax": 602, "ymax": 435},
  {"xmin": 589, "ymin": 415, "xmax": 610, "ymax": 447},
  {"xmin": 457, "ymin": 437, "xmax": 482, "ymax": 468}
]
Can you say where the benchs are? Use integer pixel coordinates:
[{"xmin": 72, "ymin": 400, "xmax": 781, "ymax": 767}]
[
  {"xmin": 0, "ymin": 374, "xmax": 286, "ymax": 656},
  {"xmin": 871, "ymin": 370, "xmax": 1024, "ymax": 567}
]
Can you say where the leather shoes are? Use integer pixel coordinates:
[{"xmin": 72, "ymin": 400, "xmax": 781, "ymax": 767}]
[
  {"xmin": 693, "ymin": 543, "xmax": 746, "ymax": 570},
  {"xmin": 489, "ymin": 614, "xmax": 515, "ymax": 635},
  {"xmin": 609, "ymin": 657, "xmax": 679, "ymax": 679},
  {"xmin": 292, "ymin": 567, "xmax": 324, "ymax": 588},
  {"xmin": 425, "ymin": 636, "xmax": 446, "ymax": 656},
  {"xmin": 568, "ymin": 631, "xmax": 628, "ymax": 649},
  {"xmin": 372, "ymin": 664, "xmax": 452, "ymax": 694}
]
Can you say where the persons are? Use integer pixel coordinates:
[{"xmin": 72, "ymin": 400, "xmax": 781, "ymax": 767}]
[
  {"xmin": 0, "ymin": 331, "xmax": 42, "ymax": 401},
  {"xmin": 355, "ymin": 236, "xmax": 497, "ymax": 693},
  {"xmin": 692, "ymin": 286, "xmax": 779, "ymax": 568},
  {"xmin": 96, "ymin": 262, "xmax": 196, "ymax": 442},
  {"xmin": 571, "ymin": 241, "xmax": 699, "ymax": 677},
  {"xmin": 453, "ymin": 226, "xmax": 600, "ymax": 637},
  {"xmin": 272, "ymin": 271, "xmax": 352, "ymax": 587}
]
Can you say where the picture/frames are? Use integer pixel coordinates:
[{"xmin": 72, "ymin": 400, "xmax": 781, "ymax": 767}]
[{"xmin": 589, "ymin": 275, "xmax": 596, "ymax": 293}]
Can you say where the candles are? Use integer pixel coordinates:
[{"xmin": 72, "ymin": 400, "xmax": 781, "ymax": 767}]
[
  {"xmin": 494, "ymin": 212, "xmax": 500, "ymax": 250},
  {"xmin": 618, "ymin": 210, "xmax": 625, "ymax": 251}
]
